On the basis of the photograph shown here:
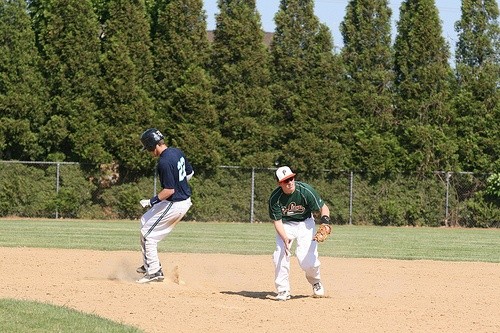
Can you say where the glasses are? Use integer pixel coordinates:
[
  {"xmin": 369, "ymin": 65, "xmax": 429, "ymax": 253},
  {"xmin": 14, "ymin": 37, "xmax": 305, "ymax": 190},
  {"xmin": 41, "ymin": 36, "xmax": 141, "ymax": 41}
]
[{"xmin": 147, "ymin": 148, "xmax": 155, "ymax": 151}]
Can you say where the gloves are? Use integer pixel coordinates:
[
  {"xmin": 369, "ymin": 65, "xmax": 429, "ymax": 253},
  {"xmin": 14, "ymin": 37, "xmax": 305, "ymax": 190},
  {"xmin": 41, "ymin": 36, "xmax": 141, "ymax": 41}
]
[{"xmin": 140, "ymin": 199, "xmax": 152, "ymax": 208}]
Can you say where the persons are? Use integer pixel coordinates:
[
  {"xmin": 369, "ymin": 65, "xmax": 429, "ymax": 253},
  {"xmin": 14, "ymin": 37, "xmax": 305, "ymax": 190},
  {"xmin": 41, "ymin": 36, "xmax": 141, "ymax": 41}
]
[
  {"xmin": 266, "ymin": 166, "xmax": 332, "ymax": 300},
  {"xmin": 135, "ymin": 128, "xmax": 192, "ymax": 284}
]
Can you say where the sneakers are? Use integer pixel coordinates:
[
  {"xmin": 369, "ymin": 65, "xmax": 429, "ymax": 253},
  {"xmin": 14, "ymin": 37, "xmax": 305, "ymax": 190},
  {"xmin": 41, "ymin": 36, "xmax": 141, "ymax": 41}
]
[
  {"xmin": 275, "ymin": 291, "xmax": 290, "ymax": 301},
  {"xmin": 313, "ymin": 282, "xmax": 324, "ymax": 297},
  {"xmin": 136, "ymin": 262, "xmax": 163, "ymax": 273},
  {"xmin": 136, "ymin": 268, "xmax": 164, "ymax": 283}
]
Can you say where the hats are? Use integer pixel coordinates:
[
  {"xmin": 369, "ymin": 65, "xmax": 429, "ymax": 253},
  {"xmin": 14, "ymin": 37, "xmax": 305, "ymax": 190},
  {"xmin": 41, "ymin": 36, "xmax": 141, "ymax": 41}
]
[
  {"xmin": 140, "ymin": 128, "xmax": 164, "ymax": 152},
  {"xmin": 275, "ymin": 166, "xmax": 296, "ymax": 183}
]
